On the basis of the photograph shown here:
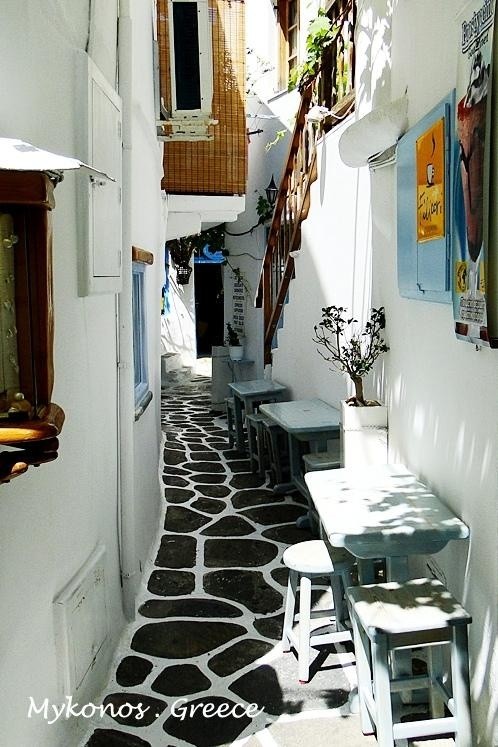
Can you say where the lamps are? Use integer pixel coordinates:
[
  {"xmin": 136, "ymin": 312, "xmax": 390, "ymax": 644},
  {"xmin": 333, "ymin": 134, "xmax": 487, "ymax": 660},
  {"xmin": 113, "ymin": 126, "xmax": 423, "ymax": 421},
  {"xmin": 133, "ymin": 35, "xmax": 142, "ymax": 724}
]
[
  {"xmin": 307, "ymin": 100, "xmax": 355, "ymax": 124},
  {"xmin": 263, "ymin": 174, "xmax": 279, "ymax": 210}
]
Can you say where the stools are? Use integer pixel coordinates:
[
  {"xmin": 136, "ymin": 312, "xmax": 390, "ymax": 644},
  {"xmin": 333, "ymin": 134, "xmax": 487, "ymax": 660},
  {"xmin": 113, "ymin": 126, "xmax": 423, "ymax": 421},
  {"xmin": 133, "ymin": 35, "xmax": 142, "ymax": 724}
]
[
  {"xmin": 225, "ymin": 396, "xmax": 341, "ymax": 532},
  {"xmin": 282, "ymin": 539, "xmax": 358, "ymax": 683},
  {"xmin": 345, "ymin": 578, "xmax": 474, "ymax": 747}
]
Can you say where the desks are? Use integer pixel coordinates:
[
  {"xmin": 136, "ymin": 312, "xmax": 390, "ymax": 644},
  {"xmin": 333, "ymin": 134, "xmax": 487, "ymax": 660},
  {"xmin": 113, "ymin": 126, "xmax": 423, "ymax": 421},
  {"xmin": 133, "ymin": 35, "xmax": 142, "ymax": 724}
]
[
  {"xmin": 304, "ymin": 463, "xmax": 471, "ymax": 705},
  {"xmin": 223, "ymin": 379, "xmax": 290, "ymax": 456}
]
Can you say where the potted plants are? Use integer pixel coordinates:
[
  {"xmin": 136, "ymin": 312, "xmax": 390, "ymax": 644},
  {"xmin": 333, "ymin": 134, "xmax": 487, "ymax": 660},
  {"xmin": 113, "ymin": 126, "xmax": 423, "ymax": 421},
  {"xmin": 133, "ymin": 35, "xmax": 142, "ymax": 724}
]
[
  {"xmin": 225, "ymin": 322, "xmax": 244, "ymax": 362},
  {"xmin": 311, "ymin": 305, "xmax": 389, "ymax": 469}
]
